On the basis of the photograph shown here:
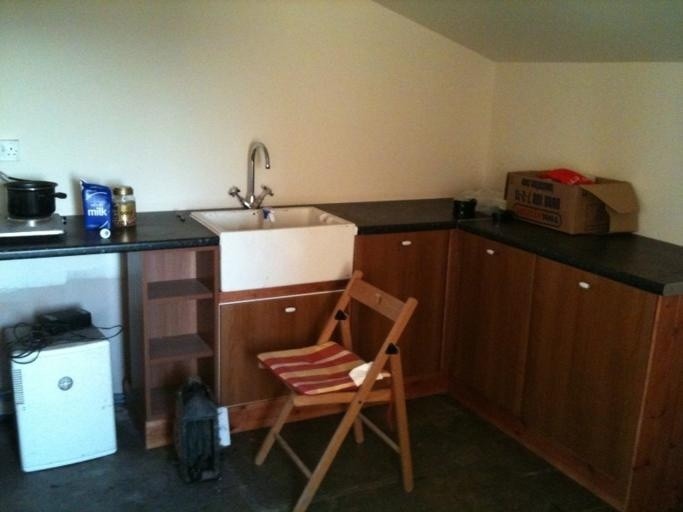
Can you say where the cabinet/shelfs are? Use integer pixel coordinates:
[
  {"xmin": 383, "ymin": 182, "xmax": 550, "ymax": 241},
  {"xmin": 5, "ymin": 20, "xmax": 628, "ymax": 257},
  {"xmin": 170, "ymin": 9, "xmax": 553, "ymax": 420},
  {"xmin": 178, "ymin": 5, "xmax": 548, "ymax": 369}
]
[
  {"xmin": 443, "ymin": 219, "xmax": 536, "ymax": 432},
  {"xmin": 526, "ymin": 235, "xmax": 683, "ymax": 511},
  {"xmin": 353, "ymin": 228, "xmax": 450, "ymax": 410},
  {"xmin": 128, "ymin": 244, "xmax": 218, "ymax": 452},
  {"xmin": 219, "ymin": 279, "xmax": 351, "ymax": 436}
]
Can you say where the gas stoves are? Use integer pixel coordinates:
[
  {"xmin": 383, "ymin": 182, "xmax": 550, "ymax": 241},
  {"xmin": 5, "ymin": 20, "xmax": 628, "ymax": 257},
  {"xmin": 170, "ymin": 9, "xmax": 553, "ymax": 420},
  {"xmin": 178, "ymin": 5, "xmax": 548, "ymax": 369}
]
[{"xmin": 0, "ymin": 213, "xmax": 66, "ymax": 247}]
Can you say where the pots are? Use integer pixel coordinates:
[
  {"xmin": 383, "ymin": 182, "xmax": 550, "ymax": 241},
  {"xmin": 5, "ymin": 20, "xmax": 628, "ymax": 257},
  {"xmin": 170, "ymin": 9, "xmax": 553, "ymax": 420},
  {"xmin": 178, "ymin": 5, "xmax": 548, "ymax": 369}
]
[{"xmin": 3, "ymin": 179, "xmax": 67, "ymax": 219}]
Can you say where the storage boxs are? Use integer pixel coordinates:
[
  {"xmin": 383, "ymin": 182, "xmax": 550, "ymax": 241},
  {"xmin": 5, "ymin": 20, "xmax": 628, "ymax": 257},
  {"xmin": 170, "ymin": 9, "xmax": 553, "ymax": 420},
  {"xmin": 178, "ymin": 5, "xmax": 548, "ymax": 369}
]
[{"xmin": 503, "ymin": 171, "xmax": 640, "ymax": 235}]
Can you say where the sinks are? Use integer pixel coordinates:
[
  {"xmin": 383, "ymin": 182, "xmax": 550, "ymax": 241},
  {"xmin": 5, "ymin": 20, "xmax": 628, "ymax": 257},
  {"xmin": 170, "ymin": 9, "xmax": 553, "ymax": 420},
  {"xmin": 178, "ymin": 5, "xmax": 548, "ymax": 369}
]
[{"xmin": 189, "ymin": 205, "xmax": 358, "ymax": 294}]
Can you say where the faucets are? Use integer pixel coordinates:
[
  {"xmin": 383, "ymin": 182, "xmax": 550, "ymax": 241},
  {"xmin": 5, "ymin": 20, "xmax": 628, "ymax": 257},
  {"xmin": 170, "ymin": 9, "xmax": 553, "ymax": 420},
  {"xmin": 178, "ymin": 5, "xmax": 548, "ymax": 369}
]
[{"xmin": 228, "ymin": 141, "xmax": 275, "ymax": 209}]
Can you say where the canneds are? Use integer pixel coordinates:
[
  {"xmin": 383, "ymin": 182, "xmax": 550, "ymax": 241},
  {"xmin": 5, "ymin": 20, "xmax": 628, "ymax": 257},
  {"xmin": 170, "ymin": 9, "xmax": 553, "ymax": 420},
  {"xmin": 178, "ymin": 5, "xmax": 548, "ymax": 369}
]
[{"xmin": 111, "ymin": 186, "xmax": 136, "ymax": 229}]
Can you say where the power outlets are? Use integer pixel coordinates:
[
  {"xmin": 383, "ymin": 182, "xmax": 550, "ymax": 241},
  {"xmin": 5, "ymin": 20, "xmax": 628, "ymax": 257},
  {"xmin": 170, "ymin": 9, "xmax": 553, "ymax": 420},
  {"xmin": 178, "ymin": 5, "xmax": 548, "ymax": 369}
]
[{"xmin": 0, "ymin": 138, "xmax": 20, "ymax": 162}]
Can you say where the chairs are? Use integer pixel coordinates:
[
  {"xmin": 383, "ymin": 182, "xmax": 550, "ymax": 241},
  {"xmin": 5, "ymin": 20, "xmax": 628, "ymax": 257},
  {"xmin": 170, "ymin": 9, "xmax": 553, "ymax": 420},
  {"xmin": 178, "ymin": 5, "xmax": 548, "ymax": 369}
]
[{"xmin": 256, "ymin": 270, "xmax": 418, "ymax": 512}]
